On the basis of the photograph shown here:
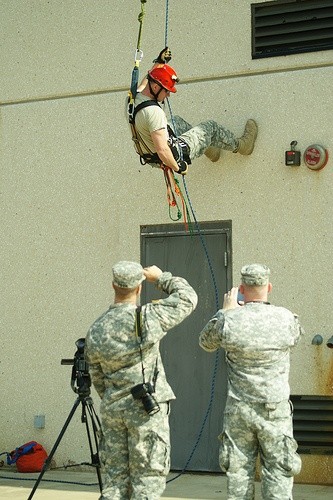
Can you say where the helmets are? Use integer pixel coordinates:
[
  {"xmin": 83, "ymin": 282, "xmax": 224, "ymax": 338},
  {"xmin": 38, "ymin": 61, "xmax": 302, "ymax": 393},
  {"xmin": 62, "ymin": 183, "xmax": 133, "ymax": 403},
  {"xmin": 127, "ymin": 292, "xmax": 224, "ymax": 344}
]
[{"xmin": 148, "ymin": 64, "xmax": 180, "ymax": 92}]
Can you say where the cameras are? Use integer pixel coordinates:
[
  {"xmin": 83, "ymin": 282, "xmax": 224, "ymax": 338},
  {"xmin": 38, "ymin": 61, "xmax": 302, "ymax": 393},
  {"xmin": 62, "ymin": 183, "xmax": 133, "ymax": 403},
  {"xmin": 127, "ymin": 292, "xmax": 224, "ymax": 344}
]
[{"xmin": 131, "ymin": 382, "xmax": 160, "ymax": 416}]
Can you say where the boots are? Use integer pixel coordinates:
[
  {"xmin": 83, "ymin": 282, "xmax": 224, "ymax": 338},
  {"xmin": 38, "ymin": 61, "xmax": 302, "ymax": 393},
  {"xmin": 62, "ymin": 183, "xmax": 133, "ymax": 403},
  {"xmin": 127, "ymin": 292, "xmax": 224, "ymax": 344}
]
[
  {"xmin": 237, "ymin": 119, "xmax": 258, "ymax": 155},
  {"xmin": 205, "ymin": 146, "xmax": 222, "ymax": 162}
]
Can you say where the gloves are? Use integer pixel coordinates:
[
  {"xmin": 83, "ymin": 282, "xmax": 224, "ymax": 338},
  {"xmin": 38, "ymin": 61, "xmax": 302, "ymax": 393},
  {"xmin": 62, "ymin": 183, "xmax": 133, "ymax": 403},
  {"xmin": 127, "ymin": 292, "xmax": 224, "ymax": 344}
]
[
  {"xmin": 153, "ymin": 47, "xmax": 172, "ymax": 64},
  {"xmin": 175, "ymin": 160, "xmax": 189, "ymax": 175}
]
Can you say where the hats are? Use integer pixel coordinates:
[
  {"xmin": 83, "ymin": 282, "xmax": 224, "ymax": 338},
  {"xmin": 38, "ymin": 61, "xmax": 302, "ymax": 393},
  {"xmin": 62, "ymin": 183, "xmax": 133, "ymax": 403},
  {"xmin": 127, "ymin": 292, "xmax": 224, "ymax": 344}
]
[
  {"xmin": 240, "ymin": 264, "xmax": 270, "ymax": 286},
  {"xmin": 111, "ymin": 260, "xmax": 146, "ymax": 289}
]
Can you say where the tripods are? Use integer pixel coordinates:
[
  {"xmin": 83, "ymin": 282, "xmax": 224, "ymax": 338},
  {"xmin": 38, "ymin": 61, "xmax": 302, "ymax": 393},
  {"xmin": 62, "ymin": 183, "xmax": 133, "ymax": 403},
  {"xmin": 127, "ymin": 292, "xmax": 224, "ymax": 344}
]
[{"xmin": 27, "ymin": 388, "xmax": 103, "ymax": 500}]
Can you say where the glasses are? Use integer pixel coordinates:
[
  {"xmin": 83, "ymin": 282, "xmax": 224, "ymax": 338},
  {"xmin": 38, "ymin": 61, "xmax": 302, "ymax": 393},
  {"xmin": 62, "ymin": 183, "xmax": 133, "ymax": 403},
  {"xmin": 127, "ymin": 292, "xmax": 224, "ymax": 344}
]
[{"xmin": 154, "ymin": 82, "xmax": 170, "ymax": 94}]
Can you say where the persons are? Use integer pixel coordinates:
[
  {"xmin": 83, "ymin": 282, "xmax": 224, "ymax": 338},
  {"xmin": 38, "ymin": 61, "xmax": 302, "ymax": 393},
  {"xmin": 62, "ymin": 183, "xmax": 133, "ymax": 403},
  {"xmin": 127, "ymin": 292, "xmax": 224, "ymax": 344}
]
[
  {"xmin": 199, "ymin": 264, "xmax": 305, "ymax": 500},
  {"xmin": 126, "ymin": 47, "xmax": 258, "ymax": 175},
  {"xmin": 85, "ymin": 261, "xmax": 197, "ymax": 500}
]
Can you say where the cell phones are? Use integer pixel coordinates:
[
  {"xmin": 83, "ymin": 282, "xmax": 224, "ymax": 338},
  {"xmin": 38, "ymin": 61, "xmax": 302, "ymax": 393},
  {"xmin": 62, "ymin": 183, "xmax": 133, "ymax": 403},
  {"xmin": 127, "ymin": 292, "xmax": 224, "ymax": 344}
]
[{"xmin": 238, "ymin": 289, "xmax": 245, "ymax": 305}]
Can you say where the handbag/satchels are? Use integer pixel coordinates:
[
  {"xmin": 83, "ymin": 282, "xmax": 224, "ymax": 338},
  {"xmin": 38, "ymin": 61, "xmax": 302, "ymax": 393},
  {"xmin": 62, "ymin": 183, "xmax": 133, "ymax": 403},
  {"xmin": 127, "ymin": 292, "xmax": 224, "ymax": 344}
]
[{"xmin": 7, "ymin": 441, "xmax": 48, "ymax": 472}]
